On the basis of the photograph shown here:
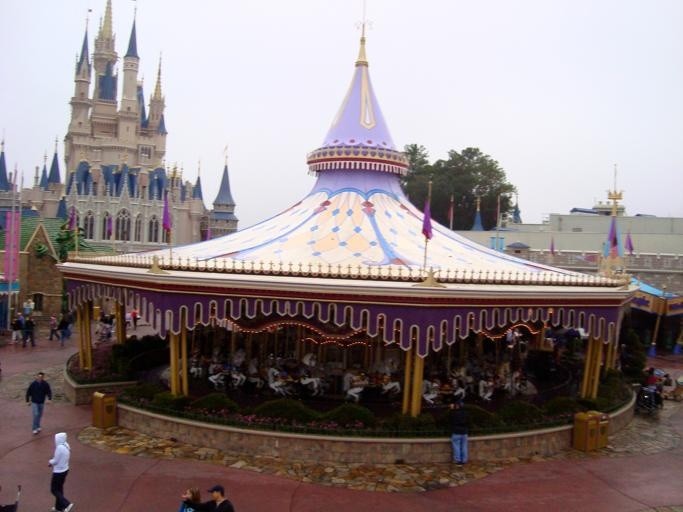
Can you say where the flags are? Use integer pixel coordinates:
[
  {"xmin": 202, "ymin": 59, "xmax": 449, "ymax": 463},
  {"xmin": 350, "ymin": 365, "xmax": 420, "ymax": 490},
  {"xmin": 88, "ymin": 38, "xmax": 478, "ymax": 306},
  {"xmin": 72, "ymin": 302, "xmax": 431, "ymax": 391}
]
[
  {"xmin": 421, "ymin": 197, "xmax": 432, "ymax": 241},
  {"xmin": 161, "ymin": 193, "xmax": 171, "ymax": 233},
  {"xmin": 623, "ymin": 234, "xmax": 634, "ymax": 251},
  {"xmin": 607, "ymin": 219, "xmax": 618, "ymax": 248},
  {"xmin": 69, "ymin": 207, "xmax": 77, "ymax": 230}
]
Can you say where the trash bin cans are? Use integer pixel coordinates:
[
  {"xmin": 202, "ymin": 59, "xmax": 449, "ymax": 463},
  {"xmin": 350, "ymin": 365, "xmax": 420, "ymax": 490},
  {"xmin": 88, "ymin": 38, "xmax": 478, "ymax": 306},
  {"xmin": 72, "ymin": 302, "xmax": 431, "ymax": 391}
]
[
  {"xmin": 93, "ymin": 307, "xmax": 99, "ymax": 320},
  {"xmin": 574, "ymin": 409, "xmax": 611, "ymax": 451},
  {"xmin": 89, "ymin": 389, "xmax": 117, "ymax": 428},
  {"xmin": 490, "ymin": 236, "xmax": 504, "ymax": 250}
]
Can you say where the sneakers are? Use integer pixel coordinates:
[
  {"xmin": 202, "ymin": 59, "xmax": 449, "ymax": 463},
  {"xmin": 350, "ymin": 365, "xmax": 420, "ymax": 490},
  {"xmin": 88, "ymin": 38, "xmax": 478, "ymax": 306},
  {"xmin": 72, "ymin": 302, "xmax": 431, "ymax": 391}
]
[{"xmin": 50, "ymin": 503, "xmax": 74, "ymax": 512}]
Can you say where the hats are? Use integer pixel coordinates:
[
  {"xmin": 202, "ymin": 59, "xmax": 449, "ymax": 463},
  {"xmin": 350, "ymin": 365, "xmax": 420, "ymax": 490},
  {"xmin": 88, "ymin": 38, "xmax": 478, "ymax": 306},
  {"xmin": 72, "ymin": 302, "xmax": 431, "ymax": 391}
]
[{"xmin": 207, "ymin": 485, "xmax": 224, "ymax": 493}]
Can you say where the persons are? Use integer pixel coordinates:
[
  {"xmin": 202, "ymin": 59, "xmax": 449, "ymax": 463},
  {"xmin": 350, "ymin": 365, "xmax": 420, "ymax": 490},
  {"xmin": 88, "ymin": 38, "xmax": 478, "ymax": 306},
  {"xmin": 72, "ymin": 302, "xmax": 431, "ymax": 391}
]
[
  {"xmin": 7, "ymin": 298, "xmax": 138, "ymax": 350},
  {"xmin": 47, "ymin": 432, "xmax": 75, "ymax": 512},
  {"xmin": 177, "ymin": 485, "xmax": 235, "ymax": 512},
  {"xmin": 447, "ymin": 400, "xmax": 469, "ymax": 465},
  {"xmin": 25, "ymin": 372, "xmax": 52, "ymax": 434},
  {"xmin": 642, "ymin": 367, "xmax": 672, "ymax": 388}
]
